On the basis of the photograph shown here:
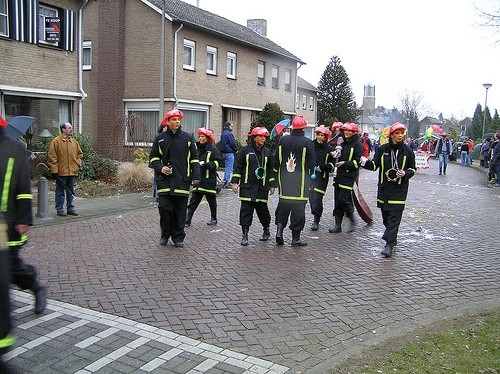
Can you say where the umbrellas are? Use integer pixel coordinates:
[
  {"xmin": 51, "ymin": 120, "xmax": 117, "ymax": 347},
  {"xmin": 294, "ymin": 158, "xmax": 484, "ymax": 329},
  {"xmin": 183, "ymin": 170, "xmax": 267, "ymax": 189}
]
[
  {"xmin": 270, "ymin": 119, "xmax": 290, "ymax": 138},
  {"xmin": 36, "ymin": 163, "xmax": 76, "ymax": 199},
  {"xmin": 2, "ymin": 115, "xmax": 35, "ymax": 141}
]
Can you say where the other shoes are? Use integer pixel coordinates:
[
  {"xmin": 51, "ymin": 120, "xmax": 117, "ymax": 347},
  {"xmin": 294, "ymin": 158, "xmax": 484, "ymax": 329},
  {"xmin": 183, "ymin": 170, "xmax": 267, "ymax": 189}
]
[
  {"xmin": 159, "ymin": 235, "xmax": 169, "ymax": 245},
  {"xmin": 67, "ymin": 210, "xmax": 78, "ymax": 216},
  {"xmin": 34, "ymin": 285, "xmax": 47, "ymax": 314},
  {"xmin": 381, "ymin": 242, "xmax": 394, "ymax": 257},
  {"xmin": 57, "ymin": 211, "xmax": 67, "ymax": 216},
  {"xmin": 487, "ymin": 178, "xmax": 500, "ymax": 187},
  {"xmin": 174, "ymin": 240, "xmax": 184, "ymax": 247}
]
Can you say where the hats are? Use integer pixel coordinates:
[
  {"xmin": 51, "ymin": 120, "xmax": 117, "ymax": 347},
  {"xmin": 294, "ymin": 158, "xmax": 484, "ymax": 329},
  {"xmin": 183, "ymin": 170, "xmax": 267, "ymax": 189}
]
[
  {"xmin": 440, "ymin": 132, "xmax": 450, "ymax": 137},
  {"xmin": 223, "ymin": 120, "xmax": 234, "ymax": 128}
]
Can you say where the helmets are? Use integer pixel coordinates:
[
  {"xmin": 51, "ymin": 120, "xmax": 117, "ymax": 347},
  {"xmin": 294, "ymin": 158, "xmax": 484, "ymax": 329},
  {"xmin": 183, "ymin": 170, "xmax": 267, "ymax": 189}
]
[
  {"xmin": 247, "ymin": 126, "xmax": 270, "ymax": 137},
  {"xmin": 342, "ymin": 121, "xmax": 359, "ymax": 132},
  {"xmin": 196, "ymin": 128, "xmax": 216, "ymax": 143},
  {"xmin": 390, "ymin": 122, "xmax": 407, "ymax": 134},
  {"xmin": 315, "ymin": 125, "xmax": 330, "ymax": 135},
  {"xmin": 161, "ymin": 109, "xmax": 183, "ymax": 126},
  {"xmin": 331, "ymin": 121, "xmax": 343, "ymax": 131},
  {"xmin": 291, "ymin": 116, "xmax": 307, "ymax": 129}
]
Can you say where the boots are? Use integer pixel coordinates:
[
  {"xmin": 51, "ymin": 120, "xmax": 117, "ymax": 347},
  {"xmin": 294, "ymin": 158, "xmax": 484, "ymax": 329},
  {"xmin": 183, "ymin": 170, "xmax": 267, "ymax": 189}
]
[
  {"xmin": 259, "ymin": 228, "xmax": 270, "ymax": 240},
  {"xmin": 276, "ymin": 222, "xmax": 286, "ymax": 245},
  {"xmin": 310, "ymin": 215, "xmax": 320, "ymax": 230},
  {"xmin": 329, "ymin": 214, "xmax": 342, "ymax": 232},
  {"xmin": 241, "ymin": 225, "xmax": 249, "ymax": 246},
  {"xmin": 291, "ymin": 230, "xmax": 308, "ymax": 246},
  {"xmin": 439, "ymin": 167, "xmax": 447, "ymax": 175},
  {"xmin": 346, "ymin": 216, "xmax": 358, "ymax": 233},
  {"xmin": 207, "ymin": 208, "xmax": 217, "ymax": 224},
  {"xmin": 184, "ymin": 208, "xmax": 196, "ymax": 226}
]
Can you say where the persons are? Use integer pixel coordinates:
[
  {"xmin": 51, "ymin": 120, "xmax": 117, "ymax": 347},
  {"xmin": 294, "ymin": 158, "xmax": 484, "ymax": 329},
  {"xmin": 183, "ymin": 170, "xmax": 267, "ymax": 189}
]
[
  {"xmin": 482, "ymin": 133, "xmax": 500, "ymax": 186},
  {"xmin": 309, "ymin": 124, "xmax": 335, "ymax": 231},
  {"xmin": 406, "ymin": 137, "xmax": 474, "ymax": 167},
  {"xmin": 435, "ymin": 132, "xmax": 453, "ymax": 175},
  {"xmin": 231, "ymin": 126, "xmax": 274, "ymax": 245},
  {"xmin": 328, "ymin": 122, "xmax": 379, "ymax": 233},
  {"xmin": 48, "ymin": 123, "xmax": 83, "ymax": 217},
  {"xmin": 361, "ymin": 122, "xmax": 416, "ymax": 258},
  {"xmin": 0, "ymin": 126, "xmax": 47, "ymax": 353},
  {"xmin": 220, "ymin": 121, "xmax": 239, "ymax": 183},
  {"xmin": 148, "ymin": 109, "xmax": 201, "ymax": 248},
  {"xmin": 272, "ymin": 117, "xmax": 315, "ymax": 246},
  {"xmin": 185, "ymin": 127, "xmax": 221, "ymax": 226}
]
